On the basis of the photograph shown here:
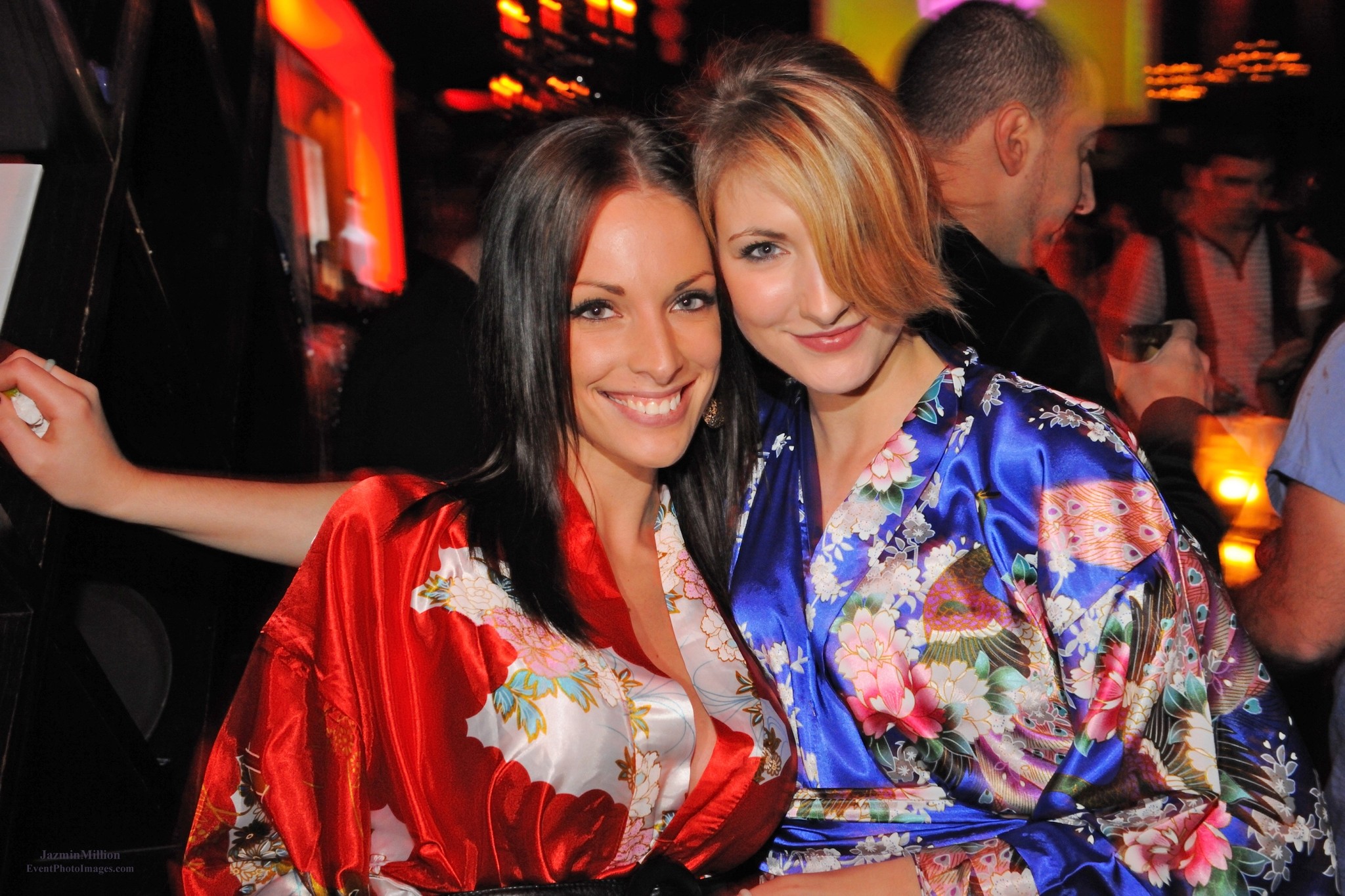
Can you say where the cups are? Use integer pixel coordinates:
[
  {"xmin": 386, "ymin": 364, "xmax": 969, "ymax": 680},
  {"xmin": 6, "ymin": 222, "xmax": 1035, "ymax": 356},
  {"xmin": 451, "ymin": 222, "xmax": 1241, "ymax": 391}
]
[{"xmin": 1113, "ymin": 321, "xmax": 1173, "ymax": 431}]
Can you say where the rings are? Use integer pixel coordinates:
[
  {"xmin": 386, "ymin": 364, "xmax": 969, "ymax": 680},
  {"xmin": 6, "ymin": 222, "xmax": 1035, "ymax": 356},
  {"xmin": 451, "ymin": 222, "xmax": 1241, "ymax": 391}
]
[{"xmin": 43, "ymin": 358, "xmax": 56, "ymax": 373}]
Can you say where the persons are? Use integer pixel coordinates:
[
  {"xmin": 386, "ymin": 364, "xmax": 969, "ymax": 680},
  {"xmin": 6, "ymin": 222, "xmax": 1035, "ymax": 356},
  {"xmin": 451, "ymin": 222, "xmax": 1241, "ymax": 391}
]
[
  {"xmin": 0, "ymin": 31, "xmax": 1344, "ymax": 896},
  {"xmin": 1097, "ymin": 132, "xmax": 1345, "ymax": 896},
  {"xmin": 179, "ymin": 109, "xmax": 799, "ymax": 895},
  {"xmin": 889, "ymin": 0, "xmax": 1230, "ymax": 576}
]
